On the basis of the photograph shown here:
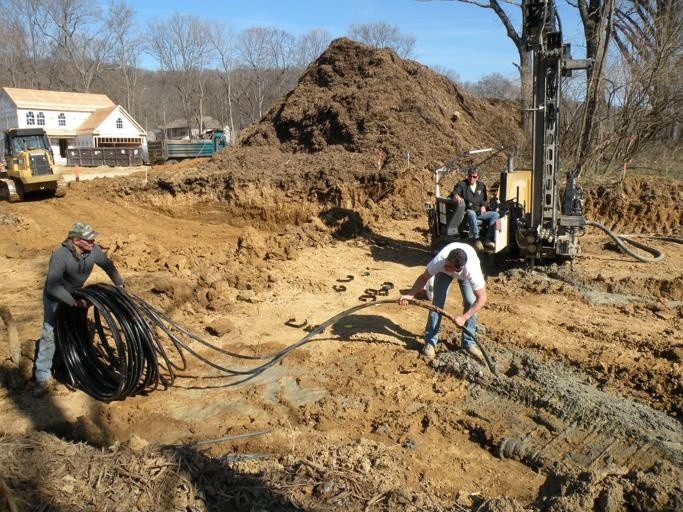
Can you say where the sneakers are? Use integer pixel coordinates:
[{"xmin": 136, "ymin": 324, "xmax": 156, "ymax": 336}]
[
  {"xmin": 462, "ymin": 345, "xmax": 481, "ymax": 358},
  {"xmin": 483, "ymin": 240, "xmax": 495, "ymax": 249},
  {"xmin": 32, "ymin": 381, "xmax": 52, "ymax": 396},
  {"xmin": 422, "ymin": 342, "xmax": 435, "ymax": 356},
  {"xmin": 473, "ymin": 240, "xmax": 484, "ymax": 251}
]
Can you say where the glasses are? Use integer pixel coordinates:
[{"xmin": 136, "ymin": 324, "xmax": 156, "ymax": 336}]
[
  {"xmin": 81, "ymin": 238, "xmax": 94, "ymax": 244},
  {"xmin": 469, "ymin": 175, "xmax": 478, "ymax": 178}
]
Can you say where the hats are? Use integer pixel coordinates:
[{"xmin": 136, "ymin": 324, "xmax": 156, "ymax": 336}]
[{"xmin": 68, "ymin": 223, "xmax": 98, "ymax": 240}]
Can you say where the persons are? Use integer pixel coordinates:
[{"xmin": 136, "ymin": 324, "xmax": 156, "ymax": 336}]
[
  {"xmin": 395, "ymin": 241, "xmax": 489, "ymax": 360},
  {"xmin": 449, "ymin": 166, "xmax": 500, "ymax": 252},
  {"xmin": 33, "ymin": 220, "xmax": 124, "ymax": 396}
]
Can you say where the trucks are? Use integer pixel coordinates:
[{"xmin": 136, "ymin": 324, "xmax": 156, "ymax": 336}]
[{"xmin": 146, "ymin": 129, "xmax": 226, "ymax": 166}]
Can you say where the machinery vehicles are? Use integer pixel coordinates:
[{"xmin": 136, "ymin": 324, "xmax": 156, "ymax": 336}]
[
  {"xmin": 0, "ymin": 126, "xmax": 66, "ymax": 204},
  {"xmin": 425, "ymin": 30, "xmax": 593, "ymax": 270}
]
